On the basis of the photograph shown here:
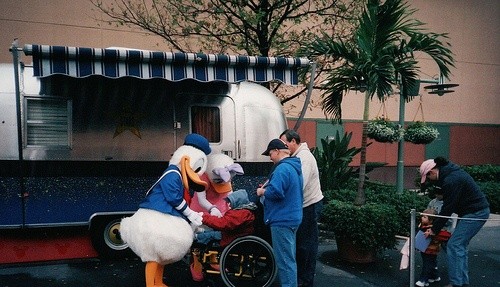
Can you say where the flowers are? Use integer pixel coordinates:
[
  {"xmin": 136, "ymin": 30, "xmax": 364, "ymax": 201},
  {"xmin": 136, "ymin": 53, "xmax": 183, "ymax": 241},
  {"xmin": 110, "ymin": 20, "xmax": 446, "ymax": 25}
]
[
  {"xmin": 368, "ymin": 115, "xmax": 403, "ymax": 142},
  {"xmin": 404, "ymin": 121, "xmax": 440, "ymax": 144}
]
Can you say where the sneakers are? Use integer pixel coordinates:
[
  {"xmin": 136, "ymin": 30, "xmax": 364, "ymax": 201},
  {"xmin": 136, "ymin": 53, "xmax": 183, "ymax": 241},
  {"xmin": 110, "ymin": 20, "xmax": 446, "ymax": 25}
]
[
  {"xmin": 428, "ymin": 277, "xmax": 441, "ymax": 283},
  {"xmin": 415, "ymin": 280, "xmax": 430, "ymax": 287}
]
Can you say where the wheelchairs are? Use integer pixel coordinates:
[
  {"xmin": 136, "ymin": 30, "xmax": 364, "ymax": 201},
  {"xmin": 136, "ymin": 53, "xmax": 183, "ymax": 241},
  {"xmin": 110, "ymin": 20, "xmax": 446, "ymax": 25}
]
[{"xmin": 189, "ymin": 180, "xmax": 278, "ymax": 287}]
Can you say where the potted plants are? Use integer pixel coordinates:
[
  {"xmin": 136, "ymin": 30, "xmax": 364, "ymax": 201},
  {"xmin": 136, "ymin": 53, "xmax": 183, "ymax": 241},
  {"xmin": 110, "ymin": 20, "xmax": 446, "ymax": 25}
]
[{"xmin": 320, "ymin": 189, "xmax": 402, "ymax": 263}]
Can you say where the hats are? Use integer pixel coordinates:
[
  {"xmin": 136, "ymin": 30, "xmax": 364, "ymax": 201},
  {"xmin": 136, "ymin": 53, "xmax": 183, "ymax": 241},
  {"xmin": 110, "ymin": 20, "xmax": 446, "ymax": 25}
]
[
  {"xmin": 261, "ymin": 139, "xmax": 288, "ymax": 156},
  {"xmin": 419, "ymin": 158, "xmax": 436, "ymax": 183}
]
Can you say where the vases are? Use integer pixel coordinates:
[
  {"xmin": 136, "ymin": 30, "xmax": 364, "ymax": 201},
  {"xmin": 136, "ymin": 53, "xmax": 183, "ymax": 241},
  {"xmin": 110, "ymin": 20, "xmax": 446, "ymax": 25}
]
[
  {"xmin": 375, "ymin": 136, "xmax": 388, "ymax": 143},
  {"xmin": 415, "ymin": 138, "xmax": 431, "ymax": 144}
]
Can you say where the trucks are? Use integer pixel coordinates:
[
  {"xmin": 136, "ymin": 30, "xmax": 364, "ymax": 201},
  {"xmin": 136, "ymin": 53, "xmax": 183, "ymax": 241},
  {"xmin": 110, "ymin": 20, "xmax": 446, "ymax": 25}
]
[{"xmin": 0, "ymin": 46, "xmax": 310, "ymax": 263}]
